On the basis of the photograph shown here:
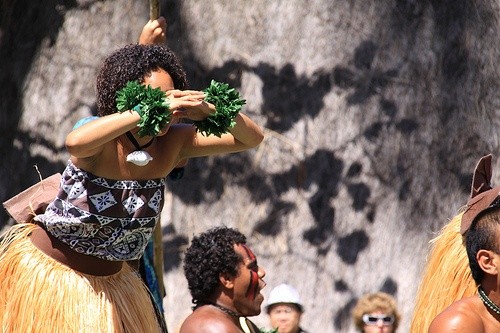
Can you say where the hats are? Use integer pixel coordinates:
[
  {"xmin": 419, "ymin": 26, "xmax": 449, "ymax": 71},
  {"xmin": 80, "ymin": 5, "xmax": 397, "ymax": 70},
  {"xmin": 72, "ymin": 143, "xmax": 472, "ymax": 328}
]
[{"xmin": 262, "ymin": 284, "xmax": 303, "ymax": 314}]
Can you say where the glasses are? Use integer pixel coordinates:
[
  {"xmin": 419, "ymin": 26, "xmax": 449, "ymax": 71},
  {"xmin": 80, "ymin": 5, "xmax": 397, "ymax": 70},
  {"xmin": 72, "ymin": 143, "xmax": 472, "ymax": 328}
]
[{"xmin": 363, "ymin": 314, "xmax": 396, "ymax": 325}]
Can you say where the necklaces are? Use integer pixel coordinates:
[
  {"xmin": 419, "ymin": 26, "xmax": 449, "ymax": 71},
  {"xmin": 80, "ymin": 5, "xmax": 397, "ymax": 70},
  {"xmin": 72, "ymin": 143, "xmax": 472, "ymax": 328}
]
[
  {"xmin": 478, "ymin": 287, "xmax": 500, "ymax": 314},
  {"xmin": 209, "ymin": 301, "xmax": 240, "ymax": 318},
  {"xmin": 126, "ymin": 131, "xmax": 155, "ymax": 167}
]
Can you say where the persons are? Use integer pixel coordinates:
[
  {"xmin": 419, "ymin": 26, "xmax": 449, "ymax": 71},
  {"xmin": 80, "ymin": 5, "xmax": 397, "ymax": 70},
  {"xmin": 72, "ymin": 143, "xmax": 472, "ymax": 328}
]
[
  {"xmin": 0, "ymin": 16, "xmax": 264, "ymax": 333},
  {"xmin": 352, "ymin": 291, "xmax": 402, "ymax": 333},
  {"xmin": 263, "ymin": 284, "xmax": 308, "ymax": 333},
  {"xmin": 179, "ymin": 222, "xmax": 266, "ymax": 333},
  {"xmin": 427, "ymin": 205, "xmax": 500, "ymax": 333}
]
